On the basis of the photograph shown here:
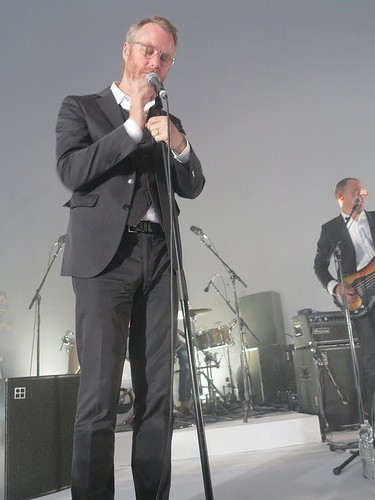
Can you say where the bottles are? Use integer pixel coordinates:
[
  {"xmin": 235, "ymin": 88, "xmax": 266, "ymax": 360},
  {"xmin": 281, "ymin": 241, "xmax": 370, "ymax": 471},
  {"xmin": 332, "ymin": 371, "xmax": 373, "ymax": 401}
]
[{"xmin": 358, "ymin": 420, "xmax": 375, "ymax": 480}]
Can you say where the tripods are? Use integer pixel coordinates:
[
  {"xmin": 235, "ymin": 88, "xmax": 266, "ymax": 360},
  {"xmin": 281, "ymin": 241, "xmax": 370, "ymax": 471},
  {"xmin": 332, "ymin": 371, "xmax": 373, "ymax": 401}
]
[
  {"xmin": 198, "ymin": 236, "xmax": 286, "ymax": 423},
  {"xmin": 327, "ymin": 203, "xmax": 365, "ymax": 475}
]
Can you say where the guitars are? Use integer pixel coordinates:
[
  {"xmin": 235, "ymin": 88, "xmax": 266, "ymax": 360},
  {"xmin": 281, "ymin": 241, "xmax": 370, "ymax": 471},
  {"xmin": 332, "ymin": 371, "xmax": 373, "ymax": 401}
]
[{"xmin": 333, "ymin": 255, "xmax": 375, "ymax": 320}]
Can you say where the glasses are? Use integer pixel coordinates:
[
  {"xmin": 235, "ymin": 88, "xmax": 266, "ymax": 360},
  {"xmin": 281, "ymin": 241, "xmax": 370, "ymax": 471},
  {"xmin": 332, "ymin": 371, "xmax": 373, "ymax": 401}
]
[
  {"xmin": 131, "ymin": 42, "xmax": 176, "ymax": 68},
  {"xmin": 344, "ymin": 189, "xmax": 369, "ymax": 200}
]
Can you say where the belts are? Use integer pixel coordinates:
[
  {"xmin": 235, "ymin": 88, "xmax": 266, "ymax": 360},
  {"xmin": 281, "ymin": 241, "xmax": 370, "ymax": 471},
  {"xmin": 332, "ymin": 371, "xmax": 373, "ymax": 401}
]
[{"xmin": 125, "ymin": 221, "xmax": 162, "ymax": 234}]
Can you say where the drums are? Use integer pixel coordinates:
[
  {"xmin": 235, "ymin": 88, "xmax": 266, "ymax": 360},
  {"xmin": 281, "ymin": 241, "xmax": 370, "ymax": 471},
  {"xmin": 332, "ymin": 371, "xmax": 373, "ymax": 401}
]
[
  {"xmin": 194, "ymin": 322, "xmax": 235, "ymax": 351},
  {"xmin": 67, "ymin": 337, "xmax": 81, "ymax": 374}
]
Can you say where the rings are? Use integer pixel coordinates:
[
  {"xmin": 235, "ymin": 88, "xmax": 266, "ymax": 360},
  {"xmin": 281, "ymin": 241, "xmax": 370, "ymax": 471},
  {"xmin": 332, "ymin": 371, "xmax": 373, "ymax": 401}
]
[{"xmin": 157, "ymin": 129, "xmax": 159, "ymax": 135}]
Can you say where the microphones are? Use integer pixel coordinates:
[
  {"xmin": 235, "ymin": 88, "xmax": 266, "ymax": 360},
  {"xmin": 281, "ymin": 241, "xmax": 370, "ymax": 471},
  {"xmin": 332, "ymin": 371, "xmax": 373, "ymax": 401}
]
[
  {"xmin": 204, "ymin": 276, "xmax": 215, "ymax": 292},
  {"xmin": 342, "ymin": 399, "xmax": 349, "ymax": 404},
  {"xmin": 190, "ymin": 226, "xmax": 206, "ymax": 240},
  {"xmin": 58, "ymin": 234, "xmax": 67, "ymax": 241},
  {"xmin": 145, "ymin": 72, "xmax": 168, "ymax": 98},
  {"xmin": 353, "ymin": 196, "xmax": 361, "ymax": 203}
]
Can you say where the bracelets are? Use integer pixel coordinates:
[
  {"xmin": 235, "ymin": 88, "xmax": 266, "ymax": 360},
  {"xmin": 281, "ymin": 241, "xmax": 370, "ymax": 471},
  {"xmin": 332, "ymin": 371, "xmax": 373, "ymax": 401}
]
[{"xmin": 173, "ymin": 134, "xmax": 186, "ymax": 155}]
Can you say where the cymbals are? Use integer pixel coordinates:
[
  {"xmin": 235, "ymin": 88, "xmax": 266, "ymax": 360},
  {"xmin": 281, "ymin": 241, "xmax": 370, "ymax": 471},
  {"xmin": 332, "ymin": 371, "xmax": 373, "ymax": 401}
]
[{"xmin": 178, "ymin": 308, "xmax": 213, "ymax": 320}]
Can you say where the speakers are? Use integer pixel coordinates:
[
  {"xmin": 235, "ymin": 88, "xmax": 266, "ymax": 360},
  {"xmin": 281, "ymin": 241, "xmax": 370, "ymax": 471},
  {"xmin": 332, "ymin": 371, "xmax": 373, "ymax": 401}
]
[
  {"xmin": 0, "ymin": 374, "xmax": 81, "ymax": 500},
  {"xmin": 236, "ymin": 290, "xmax": 369, "ymax": 430}
]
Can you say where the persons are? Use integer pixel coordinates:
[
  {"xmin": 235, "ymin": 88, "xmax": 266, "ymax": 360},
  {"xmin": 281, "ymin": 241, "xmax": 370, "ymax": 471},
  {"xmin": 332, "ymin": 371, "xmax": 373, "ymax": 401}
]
[
  {"xmin": 314, "ymin": 178, "xmax": 375, "ymax": 448},
  {"xmin": 55, "ymin": 17, "xmax": 204, "ymax": 500}
]
